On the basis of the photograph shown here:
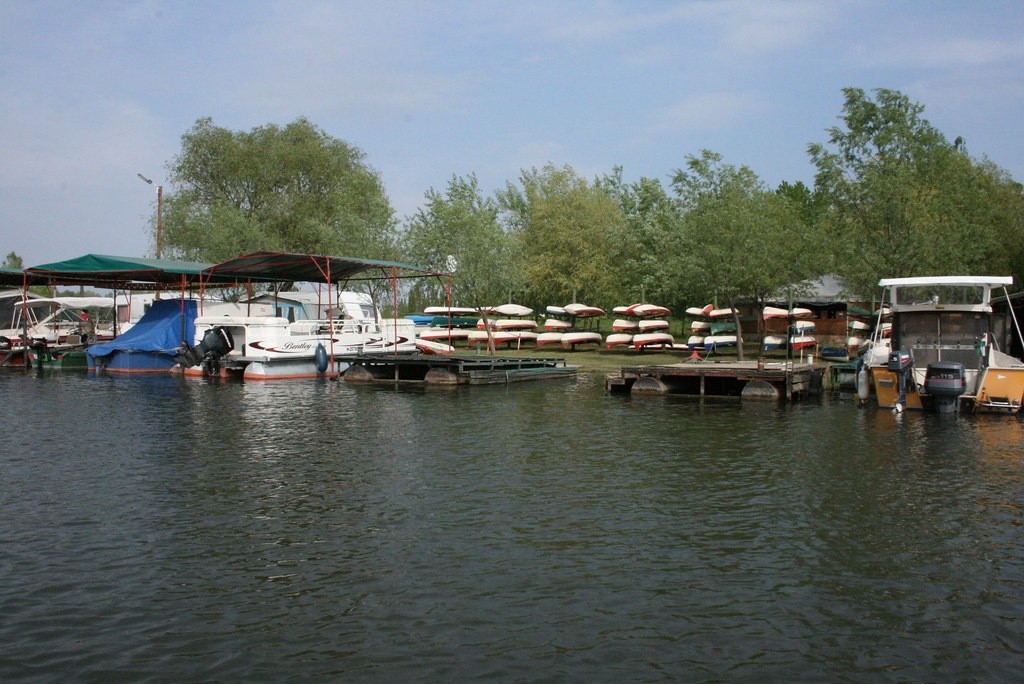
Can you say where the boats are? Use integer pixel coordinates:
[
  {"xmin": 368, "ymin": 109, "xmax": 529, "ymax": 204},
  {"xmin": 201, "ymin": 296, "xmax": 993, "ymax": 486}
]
[
  {"xmin": 536, "ymin": 306, "xmax": 605, "ymax": 350},
  {"xmin": 763, "ymin": 306, "xmax": 816, "ymax": 352},
  {"xmin": 821, "ymin": 344, "xmax": 849, "ymax": 362},
  {"xmin": 628, "ymin": 343, "xmax": 706, "ymax": 351},
  {"xmin": 856, "ymin": 276, "xmax": 1024, "ymax": 417},
  {"xmin": 846, "ymin": 307, "xmax": 892, "ymax": 354},
  {"xmin": 420, "ymin": 304, "xmax": 540, "ymax": 347},
  {"xmin": 1, "ymin": 290, "xmax": 454, "ymax": 380},
  {"xmin": 605, "ymin": 304, "xmax": 674, "ymax": 351},
  {"xmin": 686, "ymin": 307, "xmax": 744, "ymax": 353}
]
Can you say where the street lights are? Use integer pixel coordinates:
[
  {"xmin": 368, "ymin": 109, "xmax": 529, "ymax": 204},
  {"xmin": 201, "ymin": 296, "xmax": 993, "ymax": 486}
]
[{"xmin": 138, "ymin": 174, "xmax": 163, "ymax": 261}]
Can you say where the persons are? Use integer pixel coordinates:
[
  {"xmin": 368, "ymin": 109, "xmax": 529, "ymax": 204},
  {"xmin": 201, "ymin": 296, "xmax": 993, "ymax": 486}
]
[{"xmin": 80, "ymin": 309, "xmax": 89, "ymax": 321}]
